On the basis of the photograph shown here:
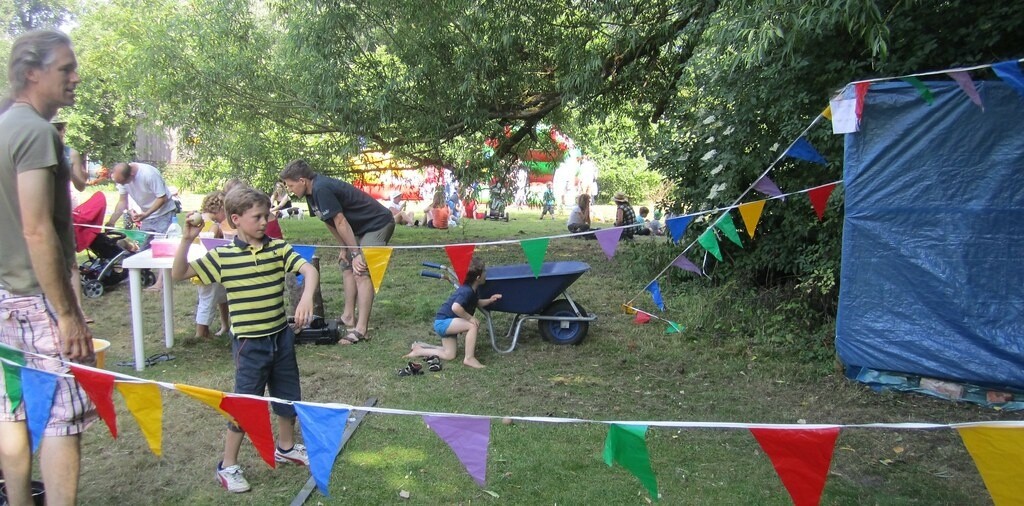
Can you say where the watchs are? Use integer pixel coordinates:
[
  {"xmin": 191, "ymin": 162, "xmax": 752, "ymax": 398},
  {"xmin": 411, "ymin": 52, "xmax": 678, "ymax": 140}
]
[{"xmin": 351, "ymin": 251, "xmax": 361, "ymax": 257}]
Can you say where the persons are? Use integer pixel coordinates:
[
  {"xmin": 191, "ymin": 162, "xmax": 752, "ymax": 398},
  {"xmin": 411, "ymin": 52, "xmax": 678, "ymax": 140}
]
[
  {"xmin": 417, "ymin": 173, "xmax": 506, "ymax": 229},
  {"xmin": 280, "ymin": 159, "xmax": 395, "ymax": 345},
  {"xmin": 610, "ymin": 190, "xmax": 636, "ymax": 241},
  {"xmin": 634, "ymin": 207, "xmax": 653, "ymax": 236},
  {"xmin": 270, "ymin": 182, "xmax": 292, "ymax": 219},
  {"xmin": 567, "ymin": 193, "xmax": 602, "ymax": 240},
  {"xmin": 172, "ymin": 189, "xmax": 319, "ymax": 492},
  {"xmin": 650, "ymin": 209, "xmax": 664, "ymax": 235},
  {"xmin": 189, "ymin": 190, "xmax": 230, "ymax": 339},
  {"xmin": 106, "ymin": 234, "xmax": 138, "ymax": 252},
  {"xmin": 171, "ymin": 191, "xmax": 183, "ymax": 223},
  {"xmin": 105, "ymin": 162, "xmax": 176, "ymax": 293},
  {"xmin": 539, "ymin": 181, "xmax": 557, "ymax": 220},
  {"xmin": 220, "ymin": 178, "xmax": 248, "ymax": 239},
  {"xmin": 0, "ymin": 30, "xmax": 94, "ymax": 506},
  {"xmin": 387, "ymin": 190, "xmax": 419, "ymax": 227},
  {"xmin": 402, "ymin": 258, "xmax": 502, "ymax": 368}
]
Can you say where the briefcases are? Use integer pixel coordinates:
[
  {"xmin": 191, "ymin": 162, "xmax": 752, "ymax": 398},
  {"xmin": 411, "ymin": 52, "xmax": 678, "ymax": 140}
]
[{"xmin": 289, "ymin": 319, "xmax": 339, "ymax": 345}]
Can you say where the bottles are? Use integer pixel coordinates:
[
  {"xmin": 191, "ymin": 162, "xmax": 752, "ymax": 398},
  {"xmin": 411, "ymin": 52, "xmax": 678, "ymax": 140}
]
[{"xmin": 167, "ymin": 218, "xmax": 181, "ymax": 241}]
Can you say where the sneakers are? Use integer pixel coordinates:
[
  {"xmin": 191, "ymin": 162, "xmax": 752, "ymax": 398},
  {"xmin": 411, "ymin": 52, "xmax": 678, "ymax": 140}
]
[
  {"xmin": 216, "ymin": 461, "xmax": 251, "ymax": 492},
  {"xmin": 274, "ymin": 443, "xmax": 310, "ymax": 466}
]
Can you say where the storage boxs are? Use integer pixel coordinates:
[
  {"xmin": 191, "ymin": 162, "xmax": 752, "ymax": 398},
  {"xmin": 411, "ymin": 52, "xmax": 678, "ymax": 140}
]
[{"xmin": 150, "ymin": 239, "xmax": 181, "ymax": 258}]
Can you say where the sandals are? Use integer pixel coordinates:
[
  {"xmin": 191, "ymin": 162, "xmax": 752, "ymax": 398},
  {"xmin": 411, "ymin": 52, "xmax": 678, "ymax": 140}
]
[
  {"xmin": 397, "ymin": 362, "xmax": 424, "ymax": 376},
  {"xmin": 426, "ymin": 355, "xmax": 441, "ymax": 371}
]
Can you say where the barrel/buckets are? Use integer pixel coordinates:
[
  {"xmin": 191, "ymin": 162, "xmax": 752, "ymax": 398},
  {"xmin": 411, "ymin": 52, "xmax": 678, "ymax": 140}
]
[{"xmin": 93, "ymin": 337, "xmax": 111, "ymax": 370}]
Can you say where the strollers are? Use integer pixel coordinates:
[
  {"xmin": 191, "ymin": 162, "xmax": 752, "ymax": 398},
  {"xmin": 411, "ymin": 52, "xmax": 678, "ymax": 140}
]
[{"xmin": 73, "ymin": 191, "xmax": 156, "ymax": 300}]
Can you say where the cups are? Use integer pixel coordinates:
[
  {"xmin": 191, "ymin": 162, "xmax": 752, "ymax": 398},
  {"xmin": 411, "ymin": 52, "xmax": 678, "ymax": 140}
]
[
  {"xmin": 153, "ymin": 234, "xmax": 166, "ymax": 239},
  {"xmin": 199, "ymin": 232, "xmax": 215, "ymax": 250}
]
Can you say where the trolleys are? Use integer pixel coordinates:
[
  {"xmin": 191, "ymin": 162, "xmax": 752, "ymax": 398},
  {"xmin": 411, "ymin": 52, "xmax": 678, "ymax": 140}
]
[{"xmin": 421, "ymin": 259, "xmax": 598, "ymax": 356}]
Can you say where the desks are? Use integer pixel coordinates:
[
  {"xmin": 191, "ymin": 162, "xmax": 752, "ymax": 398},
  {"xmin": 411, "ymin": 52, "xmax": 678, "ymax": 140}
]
[{"xmin": 122, "ymin": 244, "xmax": 208, "ymax": 367}]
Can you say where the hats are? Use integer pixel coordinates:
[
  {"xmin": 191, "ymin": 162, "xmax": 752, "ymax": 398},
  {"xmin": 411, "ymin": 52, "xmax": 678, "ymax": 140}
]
[
  {"xmin": 49, "ymin": 113, "xmax": 66, "ymax": 123},
  {"xmin": 654, "ymin": 211, "xmax": 663, "ymax": 220},
  {"xmin": 392, "ymin": 190, "xmax": 402, "ymax": 198},
  {"xmin": 612, "ymin": 192, "xmax": 628, "ymax": 202}
]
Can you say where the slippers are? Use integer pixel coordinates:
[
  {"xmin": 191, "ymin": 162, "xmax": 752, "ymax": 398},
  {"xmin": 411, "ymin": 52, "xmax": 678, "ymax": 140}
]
[
  {"xmin": 338, "ymin": 330, "xmax": 372, "ymax": 346},
  {"xmin": 116, "ymin": 356, "xmax": 151, "ymax": 367},
  {"xmin": 145, "ymin": 353, "xmax": 177, "ymax": 363},
  {"xmin": 335, "ymin": 317, "xmax": 356, "ymax": 328}
]
